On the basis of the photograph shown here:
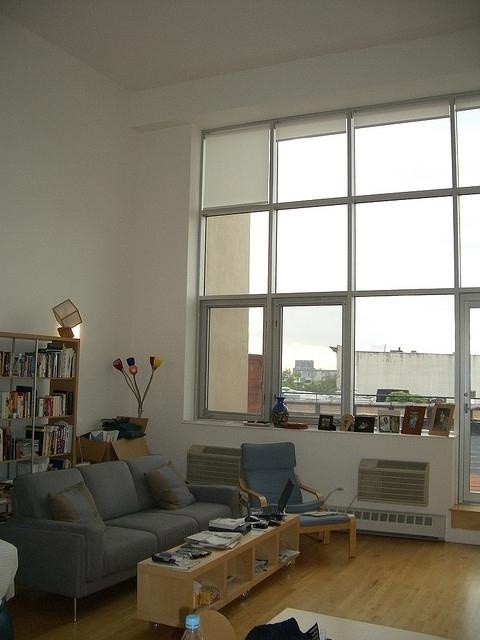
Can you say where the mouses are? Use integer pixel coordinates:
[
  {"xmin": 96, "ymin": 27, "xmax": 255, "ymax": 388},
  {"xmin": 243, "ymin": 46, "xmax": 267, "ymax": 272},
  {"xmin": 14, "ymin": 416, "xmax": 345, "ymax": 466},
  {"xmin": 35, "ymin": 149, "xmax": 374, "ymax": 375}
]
[
  {"xmin": 245, "ymin": 515, "xmax": 260, "ymax": 522},
  {"xmin": 254, "ymin": 523, "xmax": 268, "ymax": 529}
]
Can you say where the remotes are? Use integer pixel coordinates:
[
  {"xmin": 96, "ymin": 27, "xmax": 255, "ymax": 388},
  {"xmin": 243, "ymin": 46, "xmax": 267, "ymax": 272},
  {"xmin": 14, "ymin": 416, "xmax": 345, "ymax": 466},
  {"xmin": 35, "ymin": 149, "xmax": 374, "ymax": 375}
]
[{"xmin": 177, "ymin": 548, "xmax": 209, "ymax": 555}]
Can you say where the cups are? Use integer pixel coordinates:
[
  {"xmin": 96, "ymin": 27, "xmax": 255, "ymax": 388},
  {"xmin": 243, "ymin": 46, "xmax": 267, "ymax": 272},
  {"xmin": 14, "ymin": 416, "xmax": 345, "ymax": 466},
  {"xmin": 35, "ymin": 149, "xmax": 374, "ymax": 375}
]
[{"xmin": 177, "ymin": 553, "xmax": 193, "ymax": 568}]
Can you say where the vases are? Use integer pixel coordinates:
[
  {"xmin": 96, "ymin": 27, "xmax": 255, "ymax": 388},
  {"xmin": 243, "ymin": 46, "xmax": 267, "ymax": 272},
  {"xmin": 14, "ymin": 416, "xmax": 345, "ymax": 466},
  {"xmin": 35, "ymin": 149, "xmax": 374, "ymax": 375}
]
[{"xmin": 272, "ymin": 397, "xmax": 289, "ymax": 427}]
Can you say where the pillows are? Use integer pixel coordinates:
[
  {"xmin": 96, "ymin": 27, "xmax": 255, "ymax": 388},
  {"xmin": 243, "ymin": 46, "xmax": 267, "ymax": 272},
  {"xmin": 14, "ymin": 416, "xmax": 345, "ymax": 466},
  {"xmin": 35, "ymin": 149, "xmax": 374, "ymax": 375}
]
[
  {"xmin": 48, "ymin": 481, "xmax": 106, "ymax": 530},
  {"xmin": 144, "ymin": 462, "xmax": 196, "ymax": 511}
]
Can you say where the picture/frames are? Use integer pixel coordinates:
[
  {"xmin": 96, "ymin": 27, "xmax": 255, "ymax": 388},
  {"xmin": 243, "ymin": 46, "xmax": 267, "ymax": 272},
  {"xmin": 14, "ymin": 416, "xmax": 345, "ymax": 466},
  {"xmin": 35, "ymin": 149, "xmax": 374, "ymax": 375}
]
[
  {"xmin": 340, "ymin": 414, "xmax": 353, "ymax": 431},
  {"xmin": 401, "ymin": 405, "xmax": 427, "ymax": 436},
  {"xmin": 428, "ymin": 404, "xmax": 455, "ymax": 436},
  {"xmin": 379, "ymin": 415, "xmax": 392, "ymax": 432},
  {"xmin": 391, "ymin": 416, "xmax": 401, "ymax": 433},
  {"xmin": 318, "ymin": 415, "xmax": 336, "ymax": 431},
  {"xmin": 354, "ymin": 417, "xmax": 375, "ymax": 433}
]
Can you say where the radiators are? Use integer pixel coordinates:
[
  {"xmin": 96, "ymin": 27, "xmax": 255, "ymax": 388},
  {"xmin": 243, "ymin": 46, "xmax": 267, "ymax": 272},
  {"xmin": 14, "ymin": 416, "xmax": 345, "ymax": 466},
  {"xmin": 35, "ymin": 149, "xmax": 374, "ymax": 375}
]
[
  {"xmin": 358, "ymin": 458, "xmax": 430, "ymax": 507},
  {"xmin": 186, "ymin": 444, "xmax": 241, "ymax": 485}
]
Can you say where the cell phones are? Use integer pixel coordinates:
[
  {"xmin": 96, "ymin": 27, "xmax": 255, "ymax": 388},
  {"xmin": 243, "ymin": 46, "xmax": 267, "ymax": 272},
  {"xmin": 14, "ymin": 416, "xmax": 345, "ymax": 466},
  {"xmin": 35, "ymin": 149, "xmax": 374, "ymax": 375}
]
[{"xmin": 152, "ymin": 556, "xmax": 176, "ymax": 563}]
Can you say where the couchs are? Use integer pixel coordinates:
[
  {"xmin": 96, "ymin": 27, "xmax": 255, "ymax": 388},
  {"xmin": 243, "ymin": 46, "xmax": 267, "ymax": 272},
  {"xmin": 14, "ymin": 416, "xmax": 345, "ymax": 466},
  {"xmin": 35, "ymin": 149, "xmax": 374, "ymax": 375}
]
[
  {"xmin": 240, "ymin": 442, "xmax": 324, "ymax": 542},
  {"xmin": 0, "ymin": 455, "xmax": 240, "ymax": 625}
]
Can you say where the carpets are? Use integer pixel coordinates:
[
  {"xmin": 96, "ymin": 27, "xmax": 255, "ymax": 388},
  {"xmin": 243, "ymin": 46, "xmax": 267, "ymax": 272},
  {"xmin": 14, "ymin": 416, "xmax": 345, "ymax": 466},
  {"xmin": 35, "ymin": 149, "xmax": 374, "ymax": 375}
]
[{"xmin": 267, "ymin": 608, "xmax": 448, "ymax": 640}]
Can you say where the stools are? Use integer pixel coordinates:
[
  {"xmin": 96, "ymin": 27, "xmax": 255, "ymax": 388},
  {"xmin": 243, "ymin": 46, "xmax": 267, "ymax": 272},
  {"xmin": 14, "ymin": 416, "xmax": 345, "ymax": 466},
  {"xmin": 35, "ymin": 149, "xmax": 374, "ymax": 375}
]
[{"xmin": 299, "ymin": 513, "xmax": 356, "ymax": 558}]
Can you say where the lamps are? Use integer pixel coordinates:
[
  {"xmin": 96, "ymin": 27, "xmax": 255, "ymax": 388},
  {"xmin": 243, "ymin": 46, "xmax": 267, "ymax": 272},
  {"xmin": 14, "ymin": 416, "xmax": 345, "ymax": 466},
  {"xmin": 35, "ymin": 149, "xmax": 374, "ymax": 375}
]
[
  {"xmin": 52, "ymin": 299, "xmax": 82, "ymax": 338},
  {"xmin": 113, "ymin": 356, "xmax": 162, "ymax": 419}
]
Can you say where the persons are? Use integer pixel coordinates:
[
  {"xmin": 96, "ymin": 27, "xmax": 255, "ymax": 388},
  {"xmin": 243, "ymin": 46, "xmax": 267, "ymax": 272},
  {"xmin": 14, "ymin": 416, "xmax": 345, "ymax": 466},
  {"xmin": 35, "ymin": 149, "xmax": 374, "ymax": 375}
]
[
  {"xmin": 356, "ymin": 420, "xmax": 370, "ymax": 428},
  {"xmin": 407, "ymin": 414, "xmax": 418, "ymax": 429},
  {"xmin": 436, "ymin": 411, "xmax": 450, "ymax": 433},
  {"xmin": 380, "ymin": 416, "xmax": 390, "ymax": 429}
]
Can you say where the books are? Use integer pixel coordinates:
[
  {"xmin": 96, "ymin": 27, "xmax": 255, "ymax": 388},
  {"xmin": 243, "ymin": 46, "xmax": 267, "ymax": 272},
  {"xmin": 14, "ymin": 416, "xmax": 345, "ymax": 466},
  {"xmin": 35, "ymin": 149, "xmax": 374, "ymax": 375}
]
[
  {"xmin": 16, "ymin": 437, "xmax": 39, "ymax": 458},
  {"xmin": 19, "ymin": 456, "xmax": 50, "ymax": 474},
  {"xmin": 13, "ymin": 352, "xmax": 34, "ymax": 377},
  {"xmin": 2, "ymin": 510, "xmax": 11, "ymax": 522},
  {"xmin": 51, "ymin": 460, "xmax": 70, "ymax": 471},
  {"xmin": 90, "ymin": 430, "xmax": 119, "ymax": 442},
  {"xmin": 2, "ymin": 385, "xmax": 34, "ymax": 421},
  {"xmin": 281, "ymin": 547, "xmax": 301, "ymax": 564},
  {"xmin": 184, "ymin": 530, "xmax": 242, "ymax": 550},
  {"xmin": 256, "ymin": 559, "xmax": 270, "ymax": 573},
  {"xmin": 208, "ymin": 517, "xmax": 251, "ymax": 535},
  {"xmin": 37, "ymin": 390, "xmax": 73, "ymax": 416},
  {"xmin": 1, "ymin": 480, "xmax": 14, "ymax": 503},
  {"xmin": 0, "ymin": 350, "xmax": 10, "ymax": 376},
  {"xmin": 1, "ymin": 420, "xmax": 17, "ymax": 460},
  {"xmin": 38, "ymin": 343, "xmax": 76, "ymax": 379},
  {"xmin": 26, "ymin": 425, "xmax": 72, "ymax": 457}
]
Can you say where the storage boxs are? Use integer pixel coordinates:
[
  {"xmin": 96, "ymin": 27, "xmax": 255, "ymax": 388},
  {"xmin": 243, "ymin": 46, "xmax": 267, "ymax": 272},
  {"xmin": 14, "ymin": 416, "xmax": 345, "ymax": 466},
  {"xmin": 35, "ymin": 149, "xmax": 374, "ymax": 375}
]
[{"xmin": 77, "ymin": 416, "xmax": 150, "ymax": 464}]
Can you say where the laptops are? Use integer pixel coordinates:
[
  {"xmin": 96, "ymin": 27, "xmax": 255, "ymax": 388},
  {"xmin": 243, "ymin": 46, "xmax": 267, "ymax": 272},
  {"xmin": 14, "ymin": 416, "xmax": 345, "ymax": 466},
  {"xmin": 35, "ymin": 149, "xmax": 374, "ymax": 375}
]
[
  {"xmin": 257, "ymin": 477, "xmax": 295, "ymax": 518},
  {"xmin": 209, "ymin": 522, "xmax": 251, "ymax": 535}
]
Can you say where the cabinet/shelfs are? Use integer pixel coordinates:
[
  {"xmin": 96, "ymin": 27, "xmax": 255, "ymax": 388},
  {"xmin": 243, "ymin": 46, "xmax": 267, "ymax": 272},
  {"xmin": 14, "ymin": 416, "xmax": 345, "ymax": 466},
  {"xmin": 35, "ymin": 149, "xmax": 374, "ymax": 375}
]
[{"xmin": 0, "ymin": 332, "xmax": 81, "ymax": 522}]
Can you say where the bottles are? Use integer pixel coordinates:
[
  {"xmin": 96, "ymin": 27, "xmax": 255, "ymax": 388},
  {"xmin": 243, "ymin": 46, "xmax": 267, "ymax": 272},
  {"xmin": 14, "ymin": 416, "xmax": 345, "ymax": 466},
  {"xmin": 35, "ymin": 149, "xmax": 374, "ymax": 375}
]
[{"xmin": 180, "ymin": 613, "xmax": 203, "ymax": 640}]
